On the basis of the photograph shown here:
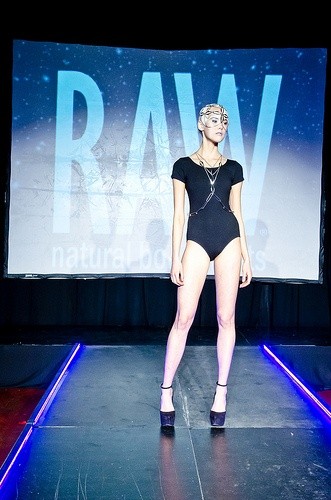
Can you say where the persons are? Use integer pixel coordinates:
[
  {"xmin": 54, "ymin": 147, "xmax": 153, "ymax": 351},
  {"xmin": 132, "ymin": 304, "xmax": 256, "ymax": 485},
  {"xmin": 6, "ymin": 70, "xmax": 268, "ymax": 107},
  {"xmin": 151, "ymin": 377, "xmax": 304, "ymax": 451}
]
[{"xmin": 159, "ymin": 104, "xmax": 251, "ymax": 427}]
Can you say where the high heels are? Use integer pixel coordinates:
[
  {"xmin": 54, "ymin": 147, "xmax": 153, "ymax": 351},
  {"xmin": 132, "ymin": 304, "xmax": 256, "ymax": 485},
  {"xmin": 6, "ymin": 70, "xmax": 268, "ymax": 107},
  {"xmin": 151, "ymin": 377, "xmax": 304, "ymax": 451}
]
[
  {"xmin": 210, "ymin": 382, "xmax": 228, "ymax": 426},
  {"xmin": 159, "ymin": 383, "xmax": 175, "ymax": 427}
]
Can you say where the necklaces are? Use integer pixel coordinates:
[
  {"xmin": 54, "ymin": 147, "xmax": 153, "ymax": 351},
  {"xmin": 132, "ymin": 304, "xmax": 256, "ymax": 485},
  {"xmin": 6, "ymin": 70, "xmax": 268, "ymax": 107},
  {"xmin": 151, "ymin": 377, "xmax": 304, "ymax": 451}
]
[{"xmin": 187, "ymin": 150, "xmax": 235, "ymax": 218}]
